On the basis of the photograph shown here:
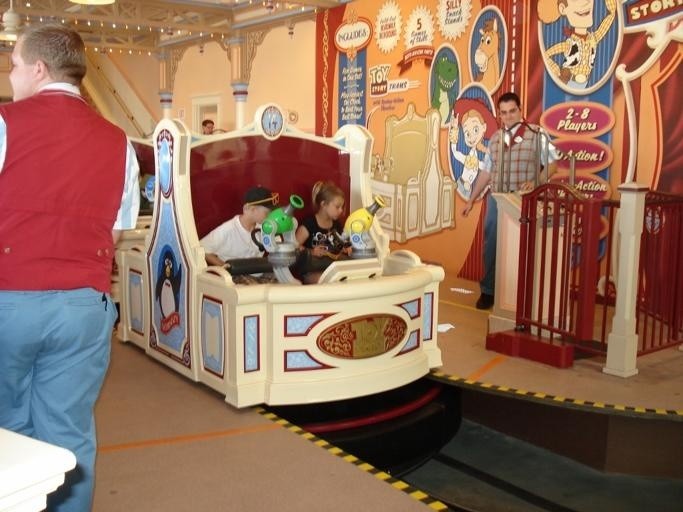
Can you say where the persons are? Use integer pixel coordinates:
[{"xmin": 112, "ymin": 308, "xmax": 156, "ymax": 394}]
[
  {"xmin": 294, "ymin": 179, "xmax": 352, "ymax": 285},
  {"xmin": 458, "ymin": 90, "xmax": 560, "ymax": 310},
  {"xmin": 199, "ymin": 186, "xmax": 277, "ymax": 285},
  {"xmin": 200, "ymin": 120, "xmax": 214, "ymax": 135},
  {"xmin": 1, "ymin": 23, "xmax": 142, "ymax": 511},
  {"xmin": 135, "ymin": 154, "xmax": 154, "ymax": 190}
]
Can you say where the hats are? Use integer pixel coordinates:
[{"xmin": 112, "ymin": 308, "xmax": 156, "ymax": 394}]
[
  {"xmin": 450, "ymin": 92, "xmax": 500, "ymax": 142},
  {"xmin": 243, "ymin": 186, "xmax": 281, "ymax": 211}
]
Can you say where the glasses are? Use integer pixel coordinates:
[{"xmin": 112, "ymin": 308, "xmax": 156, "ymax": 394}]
[{"xmin": 248, "ymin": 192, "xmax": 280, "ymax": 207}]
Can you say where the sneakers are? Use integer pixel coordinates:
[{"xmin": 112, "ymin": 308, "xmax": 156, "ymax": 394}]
[{"xmin": 475, "ymin": 292, "xmax": 495, "ymax": 310}]
